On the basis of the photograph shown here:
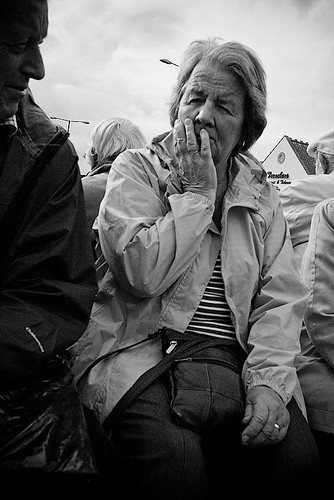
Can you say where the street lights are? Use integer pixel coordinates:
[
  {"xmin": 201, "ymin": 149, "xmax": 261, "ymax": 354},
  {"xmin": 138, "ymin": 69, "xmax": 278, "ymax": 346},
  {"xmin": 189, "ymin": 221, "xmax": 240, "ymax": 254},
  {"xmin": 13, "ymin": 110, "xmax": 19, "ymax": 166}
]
[{"xmin": 50, "ymin": 116, "xmax": 90, "ymax": 133}]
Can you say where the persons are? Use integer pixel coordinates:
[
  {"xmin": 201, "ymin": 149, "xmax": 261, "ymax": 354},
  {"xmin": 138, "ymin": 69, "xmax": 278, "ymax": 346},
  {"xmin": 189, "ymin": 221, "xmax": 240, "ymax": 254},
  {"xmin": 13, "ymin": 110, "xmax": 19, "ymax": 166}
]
[
  {"xmin": 69, "ymin": 37, "xmax": 320, "ymax": 500},
  {"xmin": 277, "ymin": 129, "xmax": 334, "ymax": 500},
  {"xmin": 0, "ymin": 0, "xmax": 99, "ymax": 500},
  {"xmin": 81, "ymin": 116, "xmax": 148, "ymax": 219}
]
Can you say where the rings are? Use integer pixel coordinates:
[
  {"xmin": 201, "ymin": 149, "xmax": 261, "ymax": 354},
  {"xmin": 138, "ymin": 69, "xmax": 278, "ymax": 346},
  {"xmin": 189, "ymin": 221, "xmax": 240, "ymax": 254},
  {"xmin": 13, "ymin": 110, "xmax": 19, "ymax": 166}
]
[{"xmin": 274, "ymin": 423, "xmax": 280, "ymax": 430}]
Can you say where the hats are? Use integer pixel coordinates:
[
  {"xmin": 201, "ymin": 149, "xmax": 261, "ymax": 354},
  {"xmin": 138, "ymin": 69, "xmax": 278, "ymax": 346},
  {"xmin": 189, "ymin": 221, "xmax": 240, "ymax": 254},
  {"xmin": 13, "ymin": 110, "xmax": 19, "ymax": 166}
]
[{"xmin": 306, "ymin": 129, "xmax": 334, "ymax": 157}]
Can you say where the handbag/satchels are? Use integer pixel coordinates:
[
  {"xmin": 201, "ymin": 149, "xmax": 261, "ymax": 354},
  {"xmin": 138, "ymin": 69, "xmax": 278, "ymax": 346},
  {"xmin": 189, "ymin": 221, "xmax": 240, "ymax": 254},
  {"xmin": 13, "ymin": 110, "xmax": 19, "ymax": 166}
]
[
  {"xmin": 156, "ymin": 324, "xmax": 248, "ymax": 437},
  {"xmin": 0, "ymin": 341, "xmax": 97, "ymax": 478}
]
